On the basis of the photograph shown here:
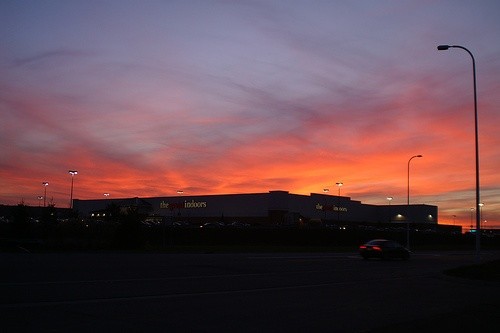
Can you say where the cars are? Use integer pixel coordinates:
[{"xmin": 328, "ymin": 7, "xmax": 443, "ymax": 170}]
[
  {"xmin": 176, "ymin": 220, "xmax": 194, "ymax": 231},
  {"xmin": 199, "ymin": 220, "xmax": 232, "ymax": 231},
  {"xmin": 358, "ymin": 238, "xmax": 412, "ymax": 261}
]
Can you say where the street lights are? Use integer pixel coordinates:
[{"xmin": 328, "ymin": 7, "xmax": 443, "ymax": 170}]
[
  {"xmin": 436, "ymin": 44, "xmax": 481, "ymax": 240},
  {"xmin": 68, "ymin": 170, "xmax": 78, "ymax": 208},
  {"xmin": 42, "ymin": 182, "xmax": 49, "ymax": 207},
  {"xmin": 177, "ymin": 191, "xmax": 184, "ymax": 217},
  {"xmin": 336, "ymin": 182, "xmax": 344, "ymax": 240},
  {"xmin": 406, "ymin": 153, "xmax": 423, "ymax": 252},
  {"xmin": 387, "ymin": 196, "xmax": 394, "ymax": 222}
]
